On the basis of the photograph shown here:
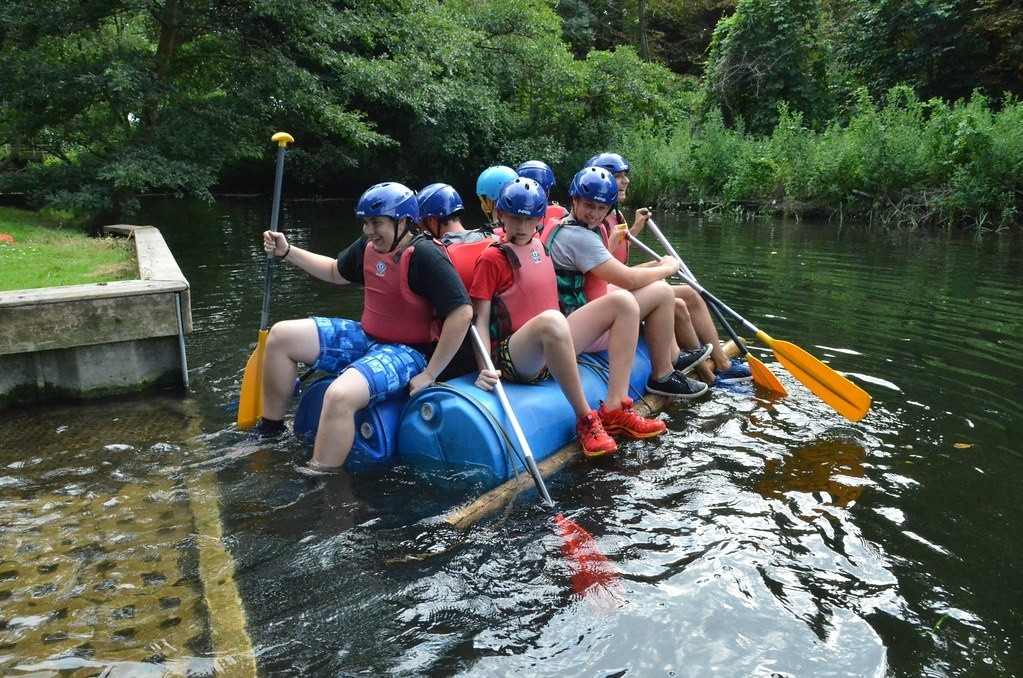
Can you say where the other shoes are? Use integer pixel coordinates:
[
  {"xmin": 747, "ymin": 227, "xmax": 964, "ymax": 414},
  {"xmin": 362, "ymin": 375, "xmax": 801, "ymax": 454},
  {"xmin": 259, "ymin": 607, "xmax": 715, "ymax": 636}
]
[
  {"xmin": 261, "ymin": 467, "xmax": 331, "ymax": 509},
  {"xmin": 233, "ymin": 424, "xmax": 291, "ymax": 449}
]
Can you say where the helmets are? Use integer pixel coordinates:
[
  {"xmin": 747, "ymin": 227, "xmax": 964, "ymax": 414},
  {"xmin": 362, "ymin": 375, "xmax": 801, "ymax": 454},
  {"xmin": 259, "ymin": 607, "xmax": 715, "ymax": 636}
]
[
  {"xmin": 568, "ymin": 166, "xmax": 618, "ymax": 206},
  {"xmin": 476, "ymin": 165, "xmax": 519, "ymax": 201},
  {"xmin": 416, "ymin": 183, "xmax": 464, "ymax": 218},
  {"xmin": 356, "ymin": 182, "xmax": 419, "ymax": 226},
  {"xmin": 516, "ymin": 160, "xmax": 556, "ymax": 197},
  {"xmin": 494, "ymin": 177, "xmax": 547, "ymax": 218},
  {"xmin": 584, "ymin": 152, "xmax": 630, "ymax": 174}
]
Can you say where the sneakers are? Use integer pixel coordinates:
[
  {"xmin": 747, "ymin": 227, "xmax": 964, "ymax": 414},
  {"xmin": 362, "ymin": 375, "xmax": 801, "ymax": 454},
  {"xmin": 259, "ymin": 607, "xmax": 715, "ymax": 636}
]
[
  {"xmin": 597, "ymin": 397, "xmax": 666, "ymax": 439},
  {"xmin": 646, "ymin": 370, "xmax": 708, "ymax": 399},
  {"xmin": 576, "ymin": 410, "xmax": 617, "ymax": 457},
  {"xmin": 714, "ymin": 358, "xmax": 754, "ymax": 384},
  {"xmin": 673, "ymin": 343, "xmax": 713, "ymax": 374}
]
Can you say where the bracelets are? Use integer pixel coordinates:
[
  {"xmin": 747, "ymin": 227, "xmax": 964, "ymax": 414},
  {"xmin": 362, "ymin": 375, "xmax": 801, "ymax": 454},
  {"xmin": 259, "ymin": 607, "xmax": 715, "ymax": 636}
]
[{"xmin": 280, "ymin": 244, "xmax": 291, "ymax": 258}]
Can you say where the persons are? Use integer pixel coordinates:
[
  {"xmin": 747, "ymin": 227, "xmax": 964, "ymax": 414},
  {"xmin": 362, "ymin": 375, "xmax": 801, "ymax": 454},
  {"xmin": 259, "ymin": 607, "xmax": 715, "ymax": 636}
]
[
  {"xmin": 415, "ymin": 183, "xmax": 501, "ymax": 289},
  {"xmin": 542, "ymin": 166, "xmax": 713, "ymax": 398},
  {"xmin": 583, "ymin": 152, "xmax": 752, "ymax": 385},
  {"xmin": 477, "ymin": 165, "xmax": 539, "ymax": 239},
  {"xmin": 249, "ymin": 182, "xmax": 474, "ymax": 466},
  {"xmin": 469, "ymin": 176, "xmax": 666, "ymax": 458},
  {"xmin": 518, "ymin": 160, "xmax": 569, "ymax": 236}
]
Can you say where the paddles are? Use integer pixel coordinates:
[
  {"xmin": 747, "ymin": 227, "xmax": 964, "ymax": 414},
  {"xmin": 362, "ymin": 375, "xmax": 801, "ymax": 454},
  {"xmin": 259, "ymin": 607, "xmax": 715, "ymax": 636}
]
[
  {"xmin": 641, "ymin": 207, "xmax": 788, "ymax": 397},
  {"xmin": 468, "ymin": 321, "xmax": 625, "ymax": 507},
  {"xmin": 237, "ymin": 132, "xmax": 295, "ymax": 424},
  {"xmin": 614, "ymin": 222, "xmax": 872, "ymax": 422}
]
